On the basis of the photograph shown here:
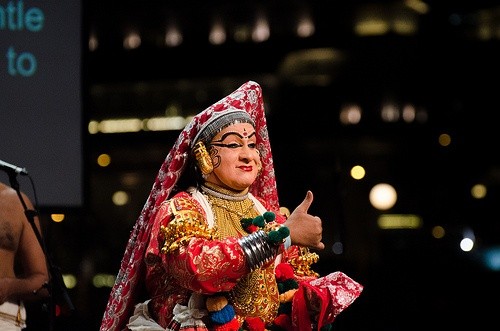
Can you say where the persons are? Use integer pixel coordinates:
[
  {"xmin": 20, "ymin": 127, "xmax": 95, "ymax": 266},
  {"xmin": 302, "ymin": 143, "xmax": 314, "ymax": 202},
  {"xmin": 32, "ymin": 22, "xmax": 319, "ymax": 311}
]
[
  {"xmin": 0, "ymin": 182, "xmax": 49, "ymax": 331},
  {"xmin": 133, "ymin": 108, "xmax": 326, "ymax": 331}
]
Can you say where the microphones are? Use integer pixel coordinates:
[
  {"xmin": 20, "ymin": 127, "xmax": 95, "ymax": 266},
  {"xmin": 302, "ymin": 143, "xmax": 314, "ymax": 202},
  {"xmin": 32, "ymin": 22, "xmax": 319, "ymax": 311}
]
[{"xmin": 0, "ymin": 159, "xmax": 29, "ymax": 175}]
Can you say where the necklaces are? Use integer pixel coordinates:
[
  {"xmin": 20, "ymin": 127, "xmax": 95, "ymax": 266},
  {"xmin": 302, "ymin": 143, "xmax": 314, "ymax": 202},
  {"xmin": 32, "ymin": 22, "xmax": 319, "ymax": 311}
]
[{"xmin": 200, "ymin": 191, "xmax": 265, "ymax": 311}]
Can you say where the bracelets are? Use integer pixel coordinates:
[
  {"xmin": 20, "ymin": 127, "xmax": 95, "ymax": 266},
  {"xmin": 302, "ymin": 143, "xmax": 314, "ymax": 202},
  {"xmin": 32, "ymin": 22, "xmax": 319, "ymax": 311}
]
[{"xmin": 239, "ymin": 220, "xmax": 289, "ymax": 271}]
